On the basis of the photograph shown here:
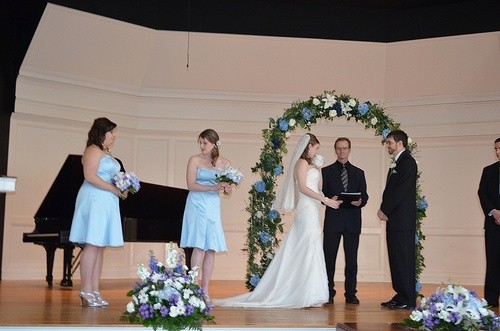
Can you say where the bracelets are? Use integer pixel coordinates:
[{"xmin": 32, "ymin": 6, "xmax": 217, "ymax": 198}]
[{"xmin": 321, "ymin": 197, "xmax": 328, "ymax": 203}]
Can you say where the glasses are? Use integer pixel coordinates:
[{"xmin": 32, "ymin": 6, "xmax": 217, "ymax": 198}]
[
  {"xmin": 386, "ymin": 141, "xmax": 396, "ymax": 145},
  {"xmin": 336, "ymin": 146, "xmax": 348, "ymax": 151}
]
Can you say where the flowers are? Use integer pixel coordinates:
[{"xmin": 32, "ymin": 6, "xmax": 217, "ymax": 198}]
[
  {"xmin": 245, "ymin": 89, "xmax": 428, "ymax": 307},
  {"xmin": 389, "ymin": 162, "xmax": 397, "ymax": 173},
  {"xmin": 110, "ymin": 171, "xmax": 141, "ymax": 200},
  {"xmin": 215, "ymin": 140, "xmax": 221, "ymax": 148},
  {"xmin": 119, "ymin": 241, "xmax": 218, "ymax": 331},
  {"xmin": 215, "ymin": 167, "xmax": 243, "ymax": 196},
  {"xmin": 402, "ymin": 285, "xmax": 500, "ymax": 331}
]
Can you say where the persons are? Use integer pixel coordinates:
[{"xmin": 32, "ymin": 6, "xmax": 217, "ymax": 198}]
[
  {"xmin": 377, "ymin": 130, "xmax": 417, "ymax": 310},
  {"xmin": 478, "ymin": 138, "xmax": 500, "ymax": 308},
  {"xmin": 69, "ymin": 117, "xmax": 128, "ymax": 307},
  {"xmin": 211, "ymin": 133, "xmax": 340, "ymax": 309},
  {"xmin": 321, "ymin": 137, "xmax": 369, "ymax": 304},
  {"xmin": 180, "ymin": 129, "xmax": 231, "ymax": 308}
]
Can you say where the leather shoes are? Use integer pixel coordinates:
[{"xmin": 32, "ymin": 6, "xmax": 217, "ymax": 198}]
[{"xmin": 380, "ymin": 299, "xmax": 416, "ymax": 310}]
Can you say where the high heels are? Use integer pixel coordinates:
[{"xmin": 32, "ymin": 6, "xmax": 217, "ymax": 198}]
[
  {"xmin": 91, "ymin": 290, "xmax": 111, "ymax": 305},
  {"xmin": 79, "ymin": 290, "xmax": 103, "ymax": 307}
]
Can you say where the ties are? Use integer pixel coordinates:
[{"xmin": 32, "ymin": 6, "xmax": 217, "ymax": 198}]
[
  {"xmin": 388, "ymin": 157, "xmax": 395, "ymax": 177},
  {"xmin": 341, "ymin": 164, "xmax": 348, "ymax": 192}
]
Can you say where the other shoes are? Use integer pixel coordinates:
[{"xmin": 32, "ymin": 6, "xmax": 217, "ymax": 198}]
[
  {"xmin": 205, "ymin": 294, "xmax": 215, "ymax": 308},
  {"xmin": 327, "ymin": 295, "xmax": 334, "ymax": 305},
  {"xmin": 345, "ymin": 296, "xmax": 360, "ymax": 304}
]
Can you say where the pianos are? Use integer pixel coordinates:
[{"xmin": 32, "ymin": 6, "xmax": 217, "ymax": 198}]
[{"xmin": 23, "ymin": 153, "xmax": 193, "ymax": 287}]
